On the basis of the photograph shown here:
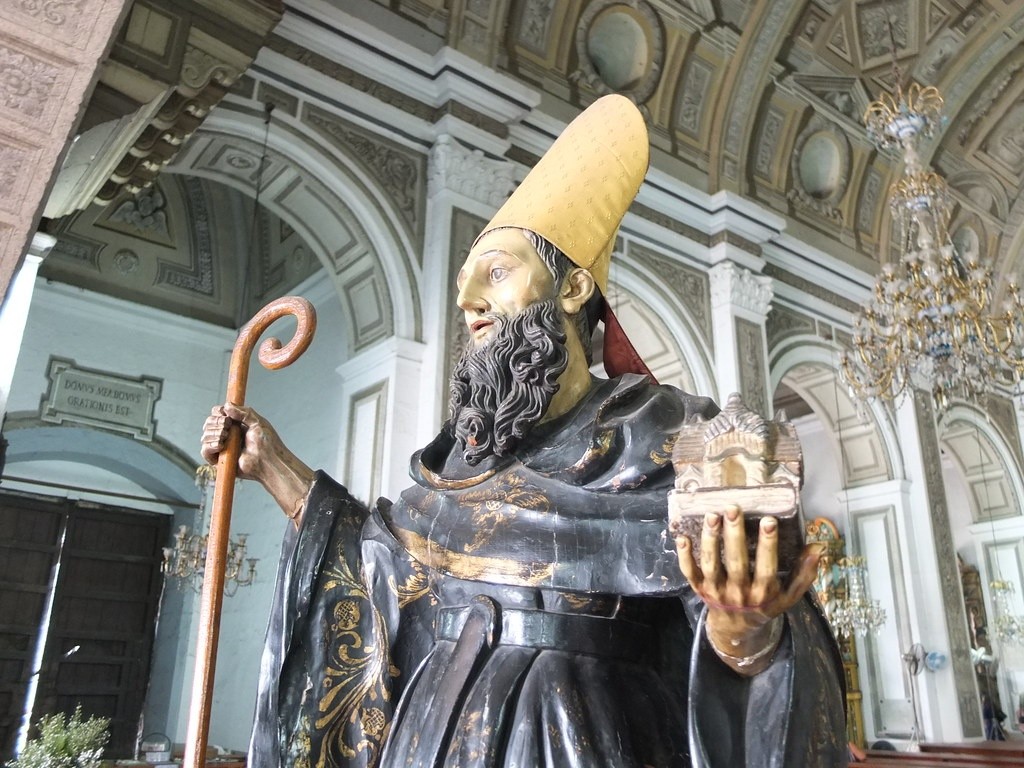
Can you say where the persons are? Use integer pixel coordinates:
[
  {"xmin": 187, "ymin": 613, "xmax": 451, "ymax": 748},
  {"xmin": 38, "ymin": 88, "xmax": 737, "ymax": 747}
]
[{"xmin": 200, "ymin": 206, "xmax": 849, "ymax": 768}]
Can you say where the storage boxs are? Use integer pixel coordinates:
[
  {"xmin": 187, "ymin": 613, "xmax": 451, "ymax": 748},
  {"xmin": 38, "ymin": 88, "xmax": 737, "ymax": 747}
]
[
  {"xmin": 141, "ymin": 742, "xmax": 168, "ymax": 752},
  {"xmin": 154, "ymin": 764, "xmax": 179, "ymax": 768},
  {"xmin": 145, "ymin": 751, "xmax": 171, "ymax": 761}
]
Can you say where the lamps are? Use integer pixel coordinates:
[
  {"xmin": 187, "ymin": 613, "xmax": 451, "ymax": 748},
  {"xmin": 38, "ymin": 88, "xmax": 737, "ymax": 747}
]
[
  {"xmin": 156, "ymin": 99, "xmax": 277, "ymax": 599},
  {"xmin": 834, "ymin": 0, "xmax": 1024, "ymax": 425},
  {"xmin": 823, "ymin": 554, "xmax": 886, "ymax": 639},
  {"xmin": 969, "ymin": 402, "xmax": 1024, "ymax": 647}
]
